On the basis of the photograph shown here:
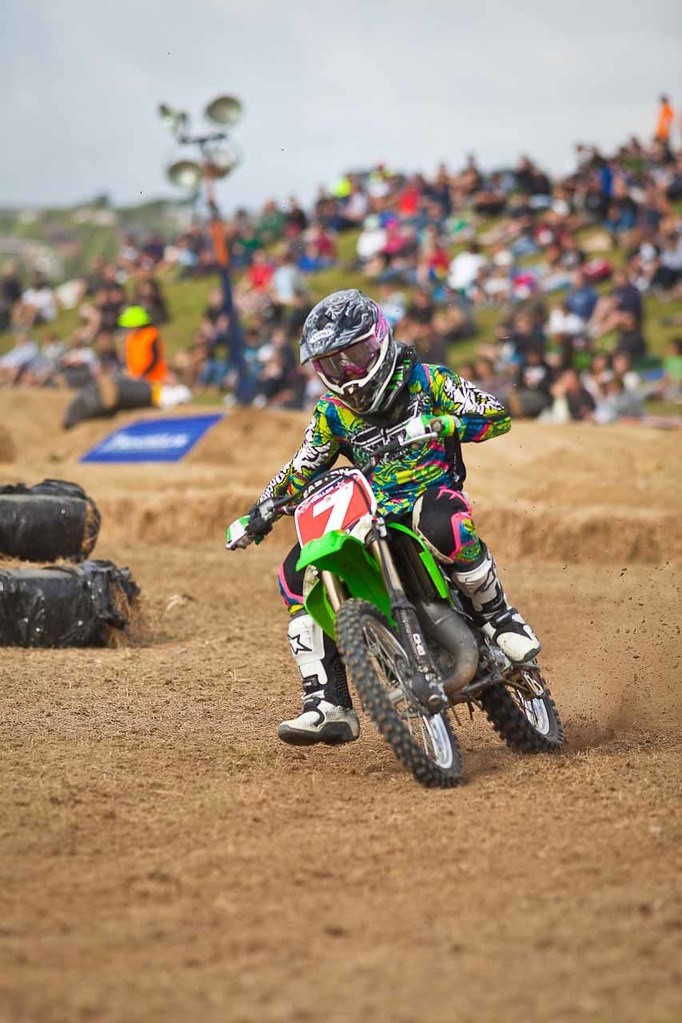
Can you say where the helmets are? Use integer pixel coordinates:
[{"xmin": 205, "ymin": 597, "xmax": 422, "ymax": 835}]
[{"xmin": 299, "ymin": 288, "xmax": 398, "ymax": 415}]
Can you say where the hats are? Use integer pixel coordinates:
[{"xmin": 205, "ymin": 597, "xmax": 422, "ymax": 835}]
[{"xmin": 117, "ymin": 306, "xmax": 152, "ymax": 328}]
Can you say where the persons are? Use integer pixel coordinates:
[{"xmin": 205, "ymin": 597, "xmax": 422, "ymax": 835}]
[
  {"xmin": 224, "ymin": 288, "xmax": 541, "ymax": 746},
  {"xmin": 656, "ymin": 95, "xmax": 673, "ymax": 140},
  {"xmin": 0, "ymin": 131, "xmax": 682, "ymax": 424}
]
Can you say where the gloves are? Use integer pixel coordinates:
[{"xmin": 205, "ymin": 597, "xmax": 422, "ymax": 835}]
[
  {"xmin": 406, "ymin": 412, "xmax": 456, "ymax": 447},
  {"xmin": 226, "ymin": 514, "xmax": 259, "ymax": 551}
]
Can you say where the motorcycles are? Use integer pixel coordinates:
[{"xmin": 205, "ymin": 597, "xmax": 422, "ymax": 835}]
[{"xmin": 222, "ymin": 412, "xmax": 564, "ymax": 790}]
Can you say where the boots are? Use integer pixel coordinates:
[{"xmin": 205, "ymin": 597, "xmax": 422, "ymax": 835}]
[
  {"xmin": 448, "ymin": 538, "xmax": 542, "ymax": 665},
  {"xmin": 276, "ymin": 614, "xmax": 360, "ymax": 746}
]
